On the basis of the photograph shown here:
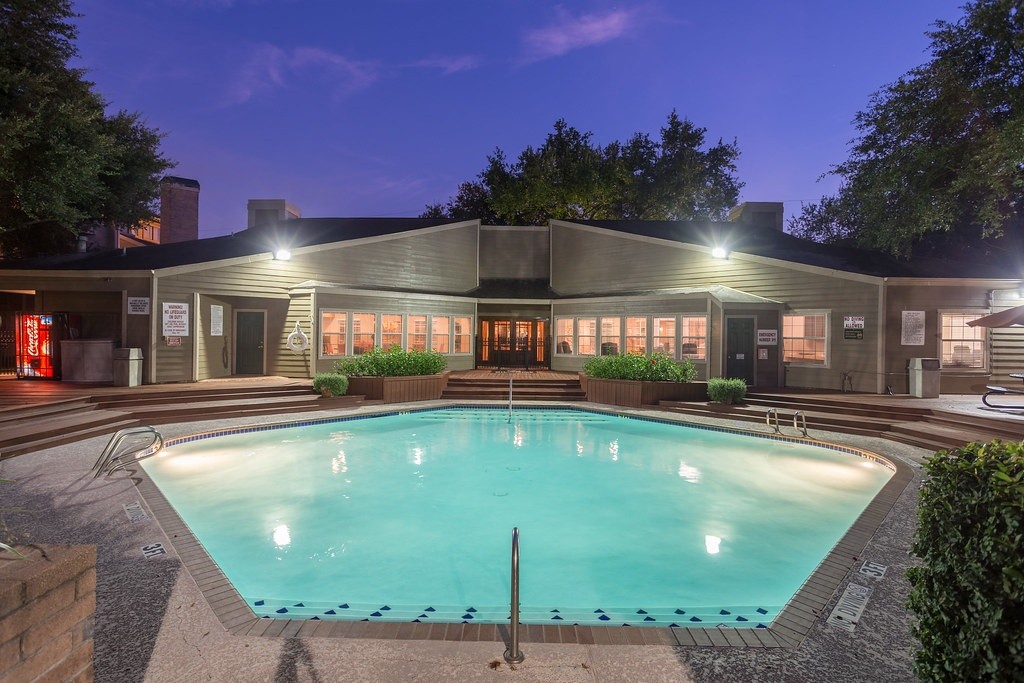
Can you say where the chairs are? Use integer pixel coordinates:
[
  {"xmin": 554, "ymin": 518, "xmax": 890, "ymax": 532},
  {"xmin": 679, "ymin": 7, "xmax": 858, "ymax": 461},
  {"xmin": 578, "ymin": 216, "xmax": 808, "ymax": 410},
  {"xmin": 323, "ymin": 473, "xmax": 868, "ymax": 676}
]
[
  {"xmin": 561, "ymin": 340, "xmax": 704, "ymax": 362},
  {"xmin": 323, "ymin": 341, "xmax": 446, "ymax": 354}
]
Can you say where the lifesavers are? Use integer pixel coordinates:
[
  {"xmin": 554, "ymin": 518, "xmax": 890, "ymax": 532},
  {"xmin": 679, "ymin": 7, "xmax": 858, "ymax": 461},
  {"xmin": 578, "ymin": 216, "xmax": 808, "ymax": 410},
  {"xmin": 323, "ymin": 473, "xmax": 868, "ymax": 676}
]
[{"xmin": 288, "ymin": 332, "xmax": 308, "ymax": 352}]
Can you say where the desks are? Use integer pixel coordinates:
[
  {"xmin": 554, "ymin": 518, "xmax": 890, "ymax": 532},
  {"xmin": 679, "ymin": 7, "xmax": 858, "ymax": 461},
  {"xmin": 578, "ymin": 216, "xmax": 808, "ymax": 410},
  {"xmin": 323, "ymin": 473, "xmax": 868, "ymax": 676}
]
[{"xmin": 1008, "ymin": 373, "xmax": 1024, "ymax": 386}]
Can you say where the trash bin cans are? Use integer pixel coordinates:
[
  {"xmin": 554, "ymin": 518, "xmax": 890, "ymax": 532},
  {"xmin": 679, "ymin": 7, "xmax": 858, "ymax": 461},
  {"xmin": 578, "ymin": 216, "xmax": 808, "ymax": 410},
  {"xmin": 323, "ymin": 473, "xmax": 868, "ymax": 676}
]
[
  {"xmin": 908, "ymin": 358, "xmax": 940, "ymax": 397},
  {"xmin": 114, "ymin": 349, "xmax": 144, "ymax": 389}
]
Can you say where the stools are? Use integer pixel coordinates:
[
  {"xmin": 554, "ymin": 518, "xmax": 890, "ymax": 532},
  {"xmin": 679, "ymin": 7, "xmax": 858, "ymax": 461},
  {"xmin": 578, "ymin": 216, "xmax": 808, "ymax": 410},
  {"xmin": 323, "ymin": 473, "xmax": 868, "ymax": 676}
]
[{"xmin": 982, "ymin": 385, "xmax": 1024, "ymax": 408}]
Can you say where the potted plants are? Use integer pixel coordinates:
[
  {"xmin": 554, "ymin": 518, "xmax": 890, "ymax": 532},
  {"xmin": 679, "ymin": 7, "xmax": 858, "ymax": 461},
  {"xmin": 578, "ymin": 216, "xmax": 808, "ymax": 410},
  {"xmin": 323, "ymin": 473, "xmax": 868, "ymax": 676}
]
[
  {"xmin": 313, "ymin": 372, "xmax": 350, "ymax": 398},
  {"xmin": 707, "ymin": 377, "xmax": 747, "ymax": 405}
]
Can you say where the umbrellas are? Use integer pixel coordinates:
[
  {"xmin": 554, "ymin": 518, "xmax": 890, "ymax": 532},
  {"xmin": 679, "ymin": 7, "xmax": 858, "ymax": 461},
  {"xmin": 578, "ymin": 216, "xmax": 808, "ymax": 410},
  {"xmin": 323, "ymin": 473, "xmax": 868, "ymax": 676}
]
[{"xmin": 966, "ymin": 305, "xmax": 1024, "ymax": 328}]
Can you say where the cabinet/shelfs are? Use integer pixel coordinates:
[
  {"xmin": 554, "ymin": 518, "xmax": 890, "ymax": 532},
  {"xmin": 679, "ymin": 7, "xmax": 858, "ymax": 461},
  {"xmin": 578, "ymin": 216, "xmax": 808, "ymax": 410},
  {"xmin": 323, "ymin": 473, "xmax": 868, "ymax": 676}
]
[{"xmin": 60, "ymin": 338, "xmax": 116, "ymax": 382}]
